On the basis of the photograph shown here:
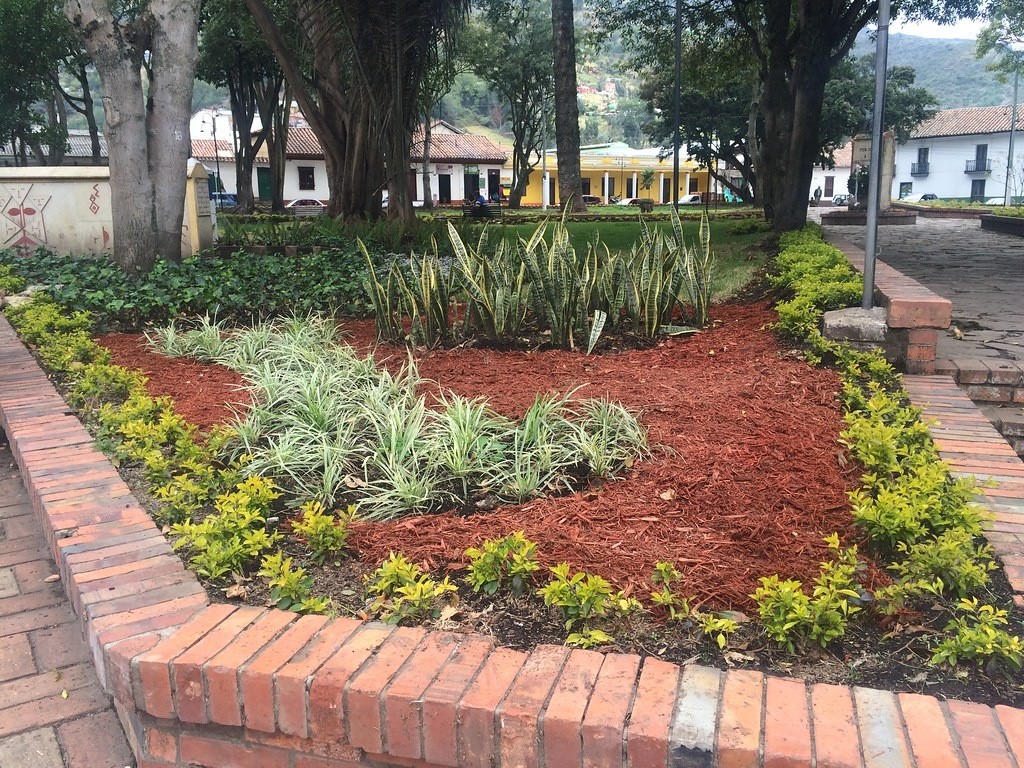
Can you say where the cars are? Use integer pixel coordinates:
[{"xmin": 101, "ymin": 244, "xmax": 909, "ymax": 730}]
[
  {"xmin": 678, "ymin": 195, "xmax": 705, "ymax": 204},
  {"xmin": 986, "ymin": 198, "xmax": 1015, "ymax": 205},
  {"xmin": 904, "ymin": 192, "xmax": 938, "ymax": 202},
  {"xmin": 616, "ymin": 198, "xmax": 640, "ymax": 205},
  {"xmin": 832, "ymin": 194, "xmax": 854, "ymax": 206},
  {"xmin": 284, "ymin": 198, "xmax": 328, "ymax": 215},
  {"xmin": 211, "ymin": 193, "xmax": 237, "ymax": 206},
  {"xmin": 582, "ymin": 195, "xmax": 603, "ymax": 206}
]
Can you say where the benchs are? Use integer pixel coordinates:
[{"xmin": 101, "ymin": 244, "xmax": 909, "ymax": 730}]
[
  {"xmin": 462, "ymin": 203, "xmax": 504, "ymax": 220},
  {"xmin": 292, "ymin": 206, "xmax": 323, "ymax": 219}
]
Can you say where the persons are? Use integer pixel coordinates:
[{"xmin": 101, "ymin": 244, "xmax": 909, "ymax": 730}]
[
  {"xmin": 814, "ymin": 186, "xmax": 822, "ymax": 204},
  {"xmin": 467, "ymin": 191, "xmax": 493, "ymax": 218},
  {"xmin": 609, "ymin": 192, "xmax": 622, "ymax": 204}
]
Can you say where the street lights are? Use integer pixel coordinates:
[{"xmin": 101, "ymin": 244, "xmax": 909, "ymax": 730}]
[{"xmin": 200, "ymin": 106, "xmax": 220, "ymax": 207}]
